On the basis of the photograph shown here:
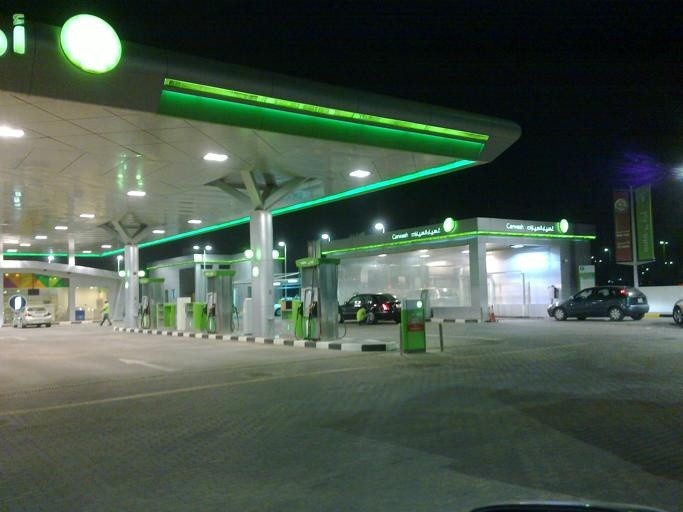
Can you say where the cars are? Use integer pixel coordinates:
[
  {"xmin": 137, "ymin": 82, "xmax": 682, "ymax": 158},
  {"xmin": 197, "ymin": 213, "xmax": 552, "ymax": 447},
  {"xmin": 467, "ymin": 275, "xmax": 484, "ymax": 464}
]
[
  {"xmin": 546, "ymin": 286, "xmax": 648, "ymax": 322},
  {"xmin": 338, "ymin": 293, "xmax": 401, "ymax": 325},
  {"xmin": 12, "ymin": 306, "xmax": 52, "ymax": 328},
  {"xmin": 672, "ymin": 299, "xmax": 682, "ymax": 324}
]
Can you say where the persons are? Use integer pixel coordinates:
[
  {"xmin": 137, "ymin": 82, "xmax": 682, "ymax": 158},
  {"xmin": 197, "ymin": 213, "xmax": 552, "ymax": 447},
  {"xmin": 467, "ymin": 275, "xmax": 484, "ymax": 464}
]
[
  {"xmin": 356, "ymin": 306, "xmax": 366, "ymax": 325},
  {"xmin": 364, "ymin": 310, "xmax": 375, "ymax": 323},
  {"xmin": 99, "ymin": 299, "xmax": 111, "ymax": 326}
]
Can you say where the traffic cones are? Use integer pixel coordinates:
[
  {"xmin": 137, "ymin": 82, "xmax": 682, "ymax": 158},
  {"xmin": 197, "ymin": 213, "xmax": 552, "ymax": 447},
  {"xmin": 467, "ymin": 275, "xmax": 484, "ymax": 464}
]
[{"xmin": 490, "ymin": 304, "xmax": 495, "ymax": 322}]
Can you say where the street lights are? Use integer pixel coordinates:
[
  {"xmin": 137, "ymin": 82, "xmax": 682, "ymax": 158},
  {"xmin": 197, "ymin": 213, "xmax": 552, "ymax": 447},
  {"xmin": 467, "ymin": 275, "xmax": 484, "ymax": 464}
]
[
  {"xmin": 116, "ymin": 255, "xmax": 123, "ymax": 273},
  {"xmin": 192, "ymin": 245, "xmax": 211, "ymax": 270},
  {"xmin": 321, "ymin": 234, "xmax": 330, "ymax": 247},
  {"xmin": 278, "ymin": 241, "xmax": 287, "ymax": 298},
  {"xmin": 374, "ymin": 223, "xmax": 384, "ymax": 234}
]
